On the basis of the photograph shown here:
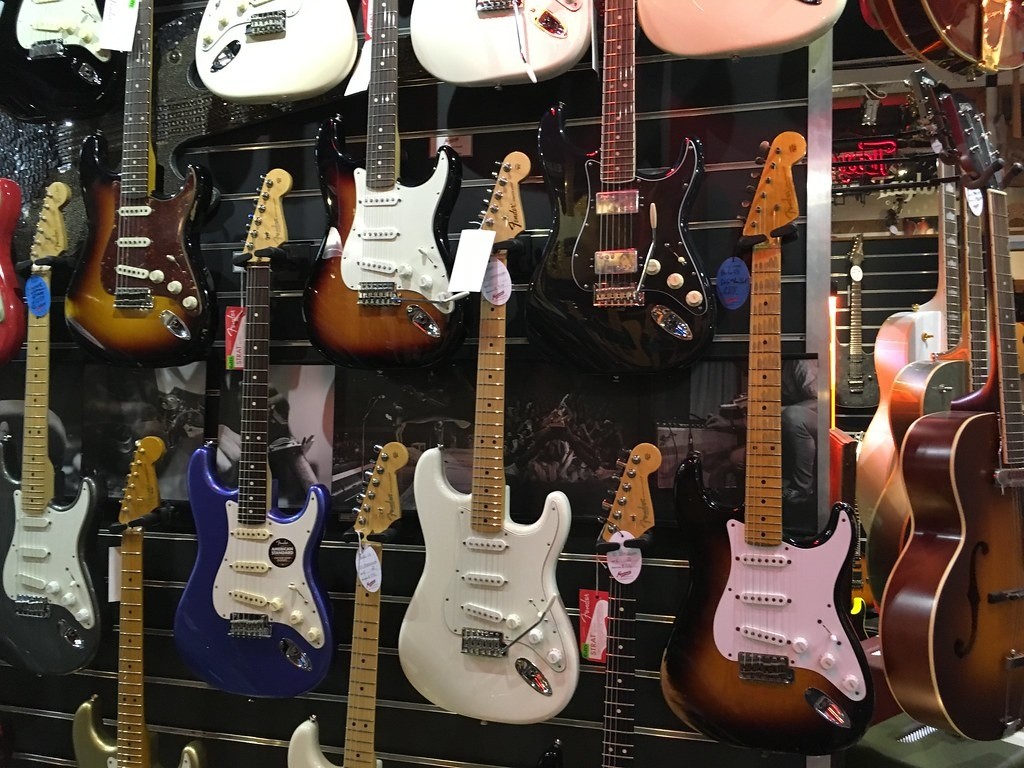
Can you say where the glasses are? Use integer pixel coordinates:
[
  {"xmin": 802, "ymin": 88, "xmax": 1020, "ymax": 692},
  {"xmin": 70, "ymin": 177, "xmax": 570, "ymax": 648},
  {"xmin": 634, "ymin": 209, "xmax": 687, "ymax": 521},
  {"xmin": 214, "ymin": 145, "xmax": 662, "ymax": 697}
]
[{"xmin": 554, "ymin": 412, "xmax": 558, "ymax": 413}]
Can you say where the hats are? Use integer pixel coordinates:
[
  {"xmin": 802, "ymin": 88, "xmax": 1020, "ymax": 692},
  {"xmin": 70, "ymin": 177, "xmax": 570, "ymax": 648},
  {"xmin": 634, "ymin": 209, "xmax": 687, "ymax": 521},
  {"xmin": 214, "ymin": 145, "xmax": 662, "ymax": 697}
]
[
  {"xmin": 552, "ymin": 409, "xmax": 559, "ymax": 412},
  {"xmin": 603, "ymin": 419, "xmax": 613, "ymax": 424}
]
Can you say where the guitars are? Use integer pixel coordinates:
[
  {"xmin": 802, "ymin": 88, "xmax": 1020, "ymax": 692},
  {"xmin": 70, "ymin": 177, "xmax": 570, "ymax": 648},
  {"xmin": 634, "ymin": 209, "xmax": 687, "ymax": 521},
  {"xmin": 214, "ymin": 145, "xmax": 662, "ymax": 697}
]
[
  {"xmin": 0, "ymin": 178, "xmax": 27, "ymax": 363},
  {"xmin": 522, "ymin": 0, "xmax": 717, "ymax": 378},
  {"xmin": 1, "ymin": 180, "xmax": 102, "ymax": 679},
  {"xmin": 73, "ymin": 434, "xmax": 208, "ymax": 768},
  {"xmin": 878, "ymin": 97, "xmax": 1023, "ymax": 743},
  {"xmin": 299, "ymin": 0, "xmax": 464, "ymax": 374},
  {"xmin": 394, "ymin": 149, "xmax": 580, "ymax": 726},
  {"xmin": 285, "ymin": 440, "xmax": 411, "ymax": 768},
  {"xmin": 834, "ymin": 231, "xmax": 880, "ymax": 410},
  {"xmin": 595, "ymin": 440, "xmax": 663, "ymax": 768},
  {"xmin": 62, "ymin": 1, "xmax": 217, "ymax": 370},
  {"xmin": 1, "ymin": 0, "xmax": 1024, "ymax": 123},
  {"xmin": 854, "ymin": 66, "xmax": 1023, "ymax": 614},
  {"xmin": 657, "ymin": 131, "xmax": 875, "ymax": 757},
  {"xmin": 172, "ymin": 167, "xmax": 337, "ymax": 701}
]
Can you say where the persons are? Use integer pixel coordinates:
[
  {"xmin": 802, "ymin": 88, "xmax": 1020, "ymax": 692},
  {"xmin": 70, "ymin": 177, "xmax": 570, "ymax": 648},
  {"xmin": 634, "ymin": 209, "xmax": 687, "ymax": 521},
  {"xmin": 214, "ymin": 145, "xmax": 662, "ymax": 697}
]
[
  {"xmin": 83, "ymin": 364, "xmax": 168, "ymax": 472},
  {"xmin": 705, "ymin": 359, "xmax": 818, "ymax": 507}
]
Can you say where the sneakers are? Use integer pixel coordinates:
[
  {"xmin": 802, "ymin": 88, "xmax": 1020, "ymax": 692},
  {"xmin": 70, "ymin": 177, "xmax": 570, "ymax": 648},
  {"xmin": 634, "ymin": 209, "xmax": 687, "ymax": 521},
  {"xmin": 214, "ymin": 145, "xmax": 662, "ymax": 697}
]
[{"xmin": 783, "ymin": 488, "xmax": 815, "ymax": 504}]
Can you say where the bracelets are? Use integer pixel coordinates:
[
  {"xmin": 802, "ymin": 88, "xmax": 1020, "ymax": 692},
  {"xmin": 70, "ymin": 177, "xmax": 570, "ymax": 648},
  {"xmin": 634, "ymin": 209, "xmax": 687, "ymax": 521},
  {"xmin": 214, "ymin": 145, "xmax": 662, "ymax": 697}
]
[{"xmin": 730, "ymin": 417, "xmax": 735, "ymax": 430}]
